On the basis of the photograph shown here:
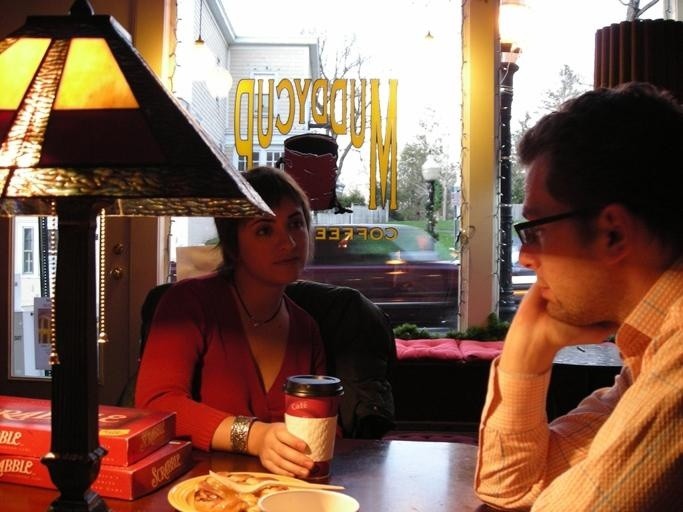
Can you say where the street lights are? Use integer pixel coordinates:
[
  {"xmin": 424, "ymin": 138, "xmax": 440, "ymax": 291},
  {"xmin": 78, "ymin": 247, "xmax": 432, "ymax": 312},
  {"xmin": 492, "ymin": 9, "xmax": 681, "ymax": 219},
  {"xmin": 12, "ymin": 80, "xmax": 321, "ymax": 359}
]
[
  {"xmin": 422, "ymin": 155, "xmax": 437, "ymax": 238},
  {"xmin": 497, "ymin": 11, "xmax": 521, "ymax": 309}
]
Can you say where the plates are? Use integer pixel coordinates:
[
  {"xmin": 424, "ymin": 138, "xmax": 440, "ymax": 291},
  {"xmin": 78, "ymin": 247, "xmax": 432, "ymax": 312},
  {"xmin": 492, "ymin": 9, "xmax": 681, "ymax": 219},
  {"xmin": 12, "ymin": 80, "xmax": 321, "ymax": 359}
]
[{"xmin": 168, "ymin": 471, "xmax": 310, "ymax": 512}]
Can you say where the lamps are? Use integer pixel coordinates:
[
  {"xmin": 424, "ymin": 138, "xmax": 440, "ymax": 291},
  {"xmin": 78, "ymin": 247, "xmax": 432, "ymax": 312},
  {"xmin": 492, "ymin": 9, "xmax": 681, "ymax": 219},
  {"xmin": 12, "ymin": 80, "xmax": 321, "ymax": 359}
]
[{"xmin": 0, "ymin": 0, "xmax": 278, "ymax": 512}]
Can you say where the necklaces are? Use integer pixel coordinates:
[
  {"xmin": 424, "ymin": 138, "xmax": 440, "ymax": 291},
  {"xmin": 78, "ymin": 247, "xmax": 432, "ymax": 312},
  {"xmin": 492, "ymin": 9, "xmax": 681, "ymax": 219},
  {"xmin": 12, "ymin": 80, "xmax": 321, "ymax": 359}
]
[{"xmin": 228, "ymin": 271, "xmax": 283, "ymax": 329}]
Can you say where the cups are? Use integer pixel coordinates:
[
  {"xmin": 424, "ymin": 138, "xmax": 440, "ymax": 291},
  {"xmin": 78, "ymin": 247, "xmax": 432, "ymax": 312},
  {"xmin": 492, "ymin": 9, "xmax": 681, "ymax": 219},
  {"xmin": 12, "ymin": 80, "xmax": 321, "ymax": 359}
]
[
  {"xmin": 284, "ymin": 374, "xmax": 345, "ymax": 482},
  {"xmin": 260, "ymin": 489, "xmax": 360, "ymax": 512}
]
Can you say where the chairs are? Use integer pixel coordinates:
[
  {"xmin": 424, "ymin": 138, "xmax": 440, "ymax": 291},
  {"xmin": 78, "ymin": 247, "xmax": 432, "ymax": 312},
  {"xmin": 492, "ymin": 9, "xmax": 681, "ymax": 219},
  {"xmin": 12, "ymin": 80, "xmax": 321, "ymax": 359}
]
[{"xmin": 142, "ymin": 281, "xmax": 393, "ymax": 438}]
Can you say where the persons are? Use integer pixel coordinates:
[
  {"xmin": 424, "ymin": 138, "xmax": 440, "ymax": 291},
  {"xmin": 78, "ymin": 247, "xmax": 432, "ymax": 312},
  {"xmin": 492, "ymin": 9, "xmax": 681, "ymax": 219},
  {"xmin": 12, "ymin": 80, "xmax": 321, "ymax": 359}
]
[
  {"xmin": 134, "ymin": 166, "xmax": 344, "ymax": 480},
  {"xmin": 471, "ymin": 81, "xmax": 680, "ymax": 512}
]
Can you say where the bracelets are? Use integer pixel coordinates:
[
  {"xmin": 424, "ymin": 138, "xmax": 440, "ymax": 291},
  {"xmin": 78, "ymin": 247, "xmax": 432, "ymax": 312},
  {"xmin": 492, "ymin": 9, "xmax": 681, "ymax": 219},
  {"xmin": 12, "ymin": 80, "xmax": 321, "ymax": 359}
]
[{"xmin": 229, "ymin": 415, "xmax": 259, "ymax": 455}]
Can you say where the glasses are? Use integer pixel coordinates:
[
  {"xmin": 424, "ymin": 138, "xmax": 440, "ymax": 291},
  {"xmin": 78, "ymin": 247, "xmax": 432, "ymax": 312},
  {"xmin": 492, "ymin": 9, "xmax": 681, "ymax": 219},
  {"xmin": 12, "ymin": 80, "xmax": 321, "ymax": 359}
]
[{"xmin": 513, "ymin": 207, "xmax": 602, "ymax": 246}]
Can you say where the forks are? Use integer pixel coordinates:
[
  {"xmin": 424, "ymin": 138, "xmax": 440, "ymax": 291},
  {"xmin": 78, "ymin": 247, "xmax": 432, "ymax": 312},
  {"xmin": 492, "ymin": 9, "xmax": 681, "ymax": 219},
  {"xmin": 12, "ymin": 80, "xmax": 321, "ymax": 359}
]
[{"xmin": 208, "ymin": 469, "xmax": 346, "ymax": 494}]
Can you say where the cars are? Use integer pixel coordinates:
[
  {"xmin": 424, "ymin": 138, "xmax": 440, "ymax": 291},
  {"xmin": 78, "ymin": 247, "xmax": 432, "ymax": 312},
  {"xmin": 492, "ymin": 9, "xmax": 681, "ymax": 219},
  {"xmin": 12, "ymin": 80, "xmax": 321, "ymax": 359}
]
[{"xmin": 297, "ymin": 223, "xmax": 461, "ymax": 325}]
